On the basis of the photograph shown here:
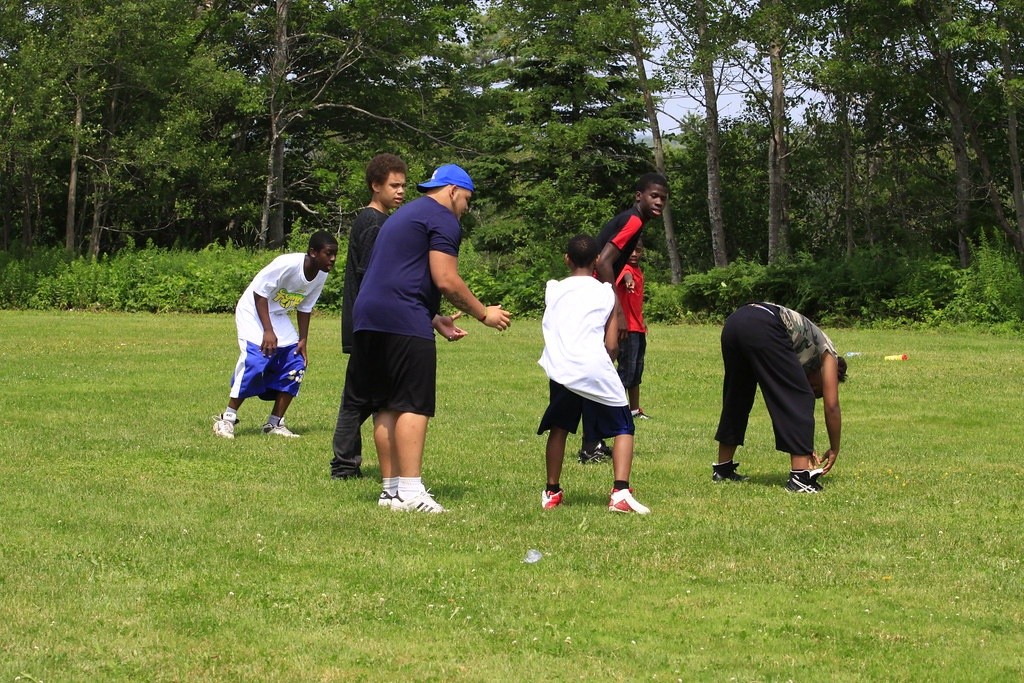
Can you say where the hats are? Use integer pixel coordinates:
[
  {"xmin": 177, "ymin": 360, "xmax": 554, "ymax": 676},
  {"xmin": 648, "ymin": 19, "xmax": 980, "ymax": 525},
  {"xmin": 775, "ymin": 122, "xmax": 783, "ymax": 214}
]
[{"xmin": 416, "ymin": 164, "xmax": 475, "ymax": 192}]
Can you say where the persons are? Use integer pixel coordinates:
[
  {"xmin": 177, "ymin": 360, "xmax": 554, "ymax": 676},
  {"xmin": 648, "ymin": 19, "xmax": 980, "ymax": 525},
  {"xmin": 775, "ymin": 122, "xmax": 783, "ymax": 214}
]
[
  {"xmin": 213, "ymin": 230, "xmax": 338, "ymax": 439},
  {"xmin": 352, "ymin": 164, "xmax": 512, "ymax": 513},
  {"xmin": 576, "ymin": 173, "xmax": 671, "ymax": 464},
  {"xmin": 330, "ymin": 151, "xmax": 408, "ymax": 479},
  {"xmin": 615, "ymin": 235, "xmax": 651, "ymax": 420},
  {"xmin": 537, "ymin": 233, "xmax": 652, "ymax": 514},
  {"xmin": 711, "ymin": 302, "xmax": 848, "ymax": 494}
]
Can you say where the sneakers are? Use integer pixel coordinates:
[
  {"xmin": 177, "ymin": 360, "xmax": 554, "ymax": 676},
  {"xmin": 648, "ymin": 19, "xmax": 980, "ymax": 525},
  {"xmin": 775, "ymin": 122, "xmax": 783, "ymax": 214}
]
[
  {"xmin": 261, "ymin": 417, "xmax": 300, "ymax": 438},
  {"xmin": 633, "ymin": 410, "xmax": 651, "ymax": 420},
  {"xmin": 579, "ymin": 442, "xmax": 616, "ymax": 464},
  {"xmin": 214, "ymin": 411, "xmax": 240, "ymax": 439},
  {"xmin": 712, "ymin": 460, "xmax": 751, "ymax": 483},
  {"xmin": 608, "ymin": 488, "xmax": 653, "ymax": 517},
  {"xmin": 785, "ymin": 468, "xmax": 824, "ymax": 494},
  {"xmin": 539, "ymin": 489, "xmax": 563, "ymax": 510},
  {"xmin": 377, "ymin": 490, "xmax": 447, "ymax": 514}
]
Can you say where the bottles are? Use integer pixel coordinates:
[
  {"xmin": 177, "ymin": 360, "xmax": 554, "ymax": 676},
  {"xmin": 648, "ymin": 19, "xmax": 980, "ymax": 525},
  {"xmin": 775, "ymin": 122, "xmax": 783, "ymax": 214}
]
[{"xmin": 884, "ymin": 354, "xmax": 908, "ymax": 361}]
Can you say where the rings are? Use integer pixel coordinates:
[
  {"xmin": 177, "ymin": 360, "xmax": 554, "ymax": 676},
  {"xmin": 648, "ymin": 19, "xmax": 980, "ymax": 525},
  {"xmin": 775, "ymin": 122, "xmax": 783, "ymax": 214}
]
[{"xmin": 448, "ymin": 336, "xmax": 452, "ymax": 341}]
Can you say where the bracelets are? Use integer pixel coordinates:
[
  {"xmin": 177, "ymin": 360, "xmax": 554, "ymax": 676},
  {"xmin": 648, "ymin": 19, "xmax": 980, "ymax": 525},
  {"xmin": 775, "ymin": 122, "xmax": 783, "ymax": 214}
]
[{"xmin": 478, "ymin": 306, "xmax": 487, "ymax": 321}]
